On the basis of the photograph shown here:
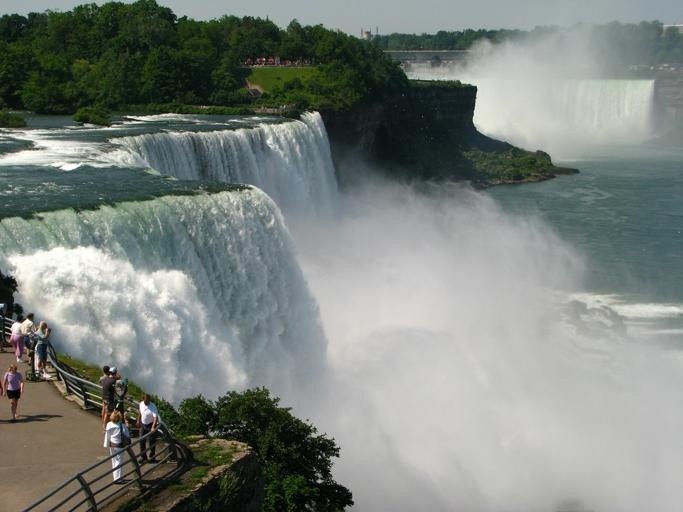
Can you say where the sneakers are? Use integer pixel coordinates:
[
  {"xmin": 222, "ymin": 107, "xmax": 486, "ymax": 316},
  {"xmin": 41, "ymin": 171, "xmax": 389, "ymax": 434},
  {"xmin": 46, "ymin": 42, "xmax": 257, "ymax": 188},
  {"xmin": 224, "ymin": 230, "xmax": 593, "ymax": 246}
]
[
  {"xmin": 43, "ymin": 373, "xmax": 51, "ymax": 378},
  {"xmin": 138, "ymin": 456, "xmax": 147, "ymax": 463}
]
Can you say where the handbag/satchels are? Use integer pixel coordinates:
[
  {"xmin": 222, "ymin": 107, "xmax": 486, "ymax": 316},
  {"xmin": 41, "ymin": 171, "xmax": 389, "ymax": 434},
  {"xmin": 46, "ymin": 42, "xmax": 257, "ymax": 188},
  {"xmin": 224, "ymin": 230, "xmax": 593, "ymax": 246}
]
[{"xmin": 119, "ymin": 434, "xmax": 131, "ymax": 446}]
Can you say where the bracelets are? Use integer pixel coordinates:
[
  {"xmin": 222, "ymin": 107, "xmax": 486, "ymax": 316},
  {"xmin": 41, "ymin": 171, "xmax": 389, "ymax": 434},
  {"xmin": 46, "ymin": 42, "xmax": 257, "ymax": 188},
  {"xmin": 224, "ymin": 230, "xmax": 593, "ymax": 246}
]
[{"xmin": 124, "ymin": 421, "xmax": 128, "ymax": 423}]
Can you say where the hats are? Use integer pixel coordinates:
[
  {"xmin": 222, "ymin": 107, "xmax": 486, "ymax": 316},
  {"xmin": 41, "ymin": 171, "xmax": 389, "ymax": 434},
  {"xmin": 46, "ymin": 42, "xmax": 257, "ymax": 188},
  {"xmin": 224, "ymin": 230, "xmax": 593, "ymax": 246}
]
[{"xmin": 109, "ymin": 366, "xmax": 117, "ymax": 372}]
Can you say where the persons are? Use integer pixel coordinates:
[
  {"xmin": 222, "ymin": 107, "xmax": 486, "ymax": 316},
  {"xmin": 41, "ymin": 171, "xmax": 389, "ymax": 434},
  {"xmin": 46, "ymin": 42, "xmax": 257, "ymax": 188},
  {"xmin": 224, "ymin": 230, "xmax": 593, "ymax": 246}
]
[
  {"xmin": 102, "ymin": 409, "xmax": 130, "ymax": 484},
  {"xmin": 0, "ymin": 379, "xmax": 2, "ymax": 396},
  {"xmin": 135, "ymin": 393, "xmax": 158, "ymax": 463},
  {"xmin": 31, "ymin": 320, "xmax": 52, "ymax": 378},
  {"xmin": 98, "ymin": 365, "xmax": 120, "ymax": 431},
  {"xmin": 9, "ymin": 314, "xmax": 27, "ymax": 364},
  {"xmin": 20, "ymin": 312, "xmax": 34, "ymax": 357},
  {"xmin": 2, "ymin": 364, "xmax": 23, "ymax": 420}
]
